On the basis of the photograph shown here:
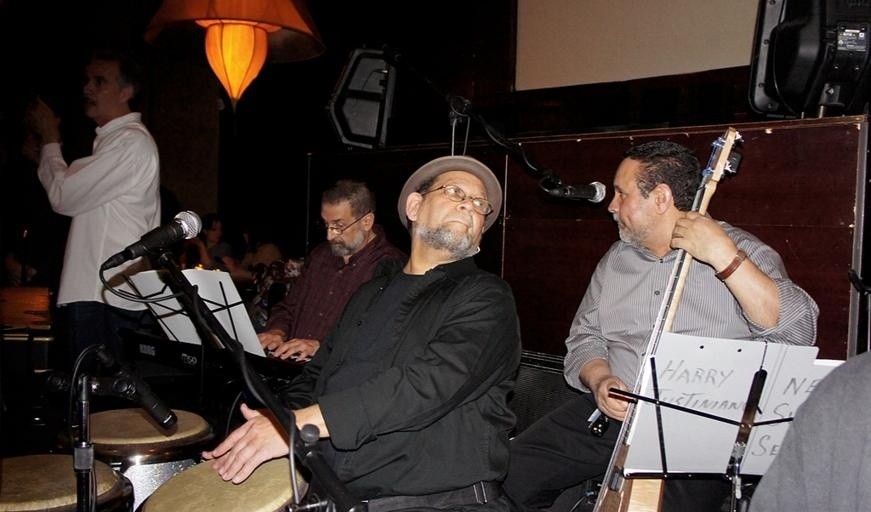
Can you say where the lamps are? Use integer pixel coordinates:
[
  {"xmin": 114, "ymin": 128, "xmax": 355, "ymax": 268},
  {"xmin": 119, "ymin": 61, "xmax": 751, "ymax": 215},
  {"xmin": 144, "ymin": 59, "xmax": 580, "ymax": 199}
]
[{"xmin": 145, "ymin": 0, "xmax": 323, "ymax": 116}]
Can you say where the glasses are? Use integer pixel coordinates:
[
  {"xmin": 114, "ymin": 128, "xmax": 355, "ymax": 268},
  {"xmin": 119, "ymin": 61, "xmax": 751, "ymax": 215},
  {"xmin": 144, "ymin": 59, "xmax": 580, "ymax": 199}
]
[
  {"xmin": 324, "ymin": 210, "xmax": 374, "ymax": 238},
  {"xmin": 422, "ymin": 184, "xmax": 494, "ymax": 217}
]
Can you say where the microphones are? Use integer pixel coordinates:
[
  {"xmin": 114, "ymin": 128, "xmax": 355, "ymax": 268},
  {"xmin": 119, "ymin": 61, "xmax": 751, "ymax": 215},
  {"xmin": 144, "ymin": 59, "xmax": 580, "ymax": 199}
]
[
  {"xmin": 548, "ymin": 179, "xmax": 610, "ymax": 209},
  {"xmin": 114, "ymin": 360, "xmax": 179, "ymax": 434},
  {"xmin": 128, "ymin": 204, "xmax": 205, "ymax": 271}
]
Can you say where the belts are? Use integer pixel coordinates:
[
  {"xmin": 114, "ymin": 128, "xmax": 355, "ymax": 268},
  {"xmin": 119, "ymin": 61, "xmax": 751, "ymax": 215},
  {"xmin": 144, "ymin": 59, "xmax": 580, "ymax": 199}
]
[{"xmin": 366, "ymin": 480, "xmax": 501, "ymax": 512}]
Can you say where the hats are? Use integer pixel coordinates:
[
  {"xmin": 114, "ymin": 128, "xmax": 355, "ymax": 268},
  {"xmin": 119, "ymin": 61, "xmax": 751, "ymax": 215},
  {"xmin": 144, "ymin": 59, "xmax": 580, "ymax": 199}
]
[{"xmin": 398, "ymin": 155, "xmax": 502, "ymax": 233}]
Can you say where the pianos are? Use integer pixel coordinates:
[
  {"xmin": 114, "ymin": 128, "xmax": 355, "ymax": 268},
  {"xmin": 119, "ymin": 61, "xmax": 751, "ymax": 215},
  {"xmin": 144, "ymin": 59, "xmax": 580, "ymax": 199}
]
[{"xmin": 119, "ymin": 327, "xmax": 315, "ymax": 382}]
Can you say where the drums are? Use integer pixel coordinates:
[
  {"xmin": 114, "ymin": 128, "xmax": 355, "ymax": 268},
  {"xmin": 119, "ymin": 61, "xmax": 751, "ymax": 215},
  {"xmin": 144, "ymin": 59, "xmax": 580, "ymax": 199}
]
[
  {"xmin": 72, "ymin": 410, "xmax": 212, "ymax": 511},
  {"xmin": 2, "ymin": 454, "xmax": 133, "ymax": 511},
  {"xmin": 135, "ymin": 458, "xmax": 307, "ymax": 512}
]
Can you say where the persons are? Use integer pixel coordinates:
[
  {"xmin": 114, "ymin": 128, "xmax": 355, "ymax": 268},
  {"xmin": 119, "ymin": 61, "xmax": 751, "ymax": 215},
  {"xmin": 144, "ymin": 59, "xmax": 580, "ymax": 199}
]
[
  {"xmin": 498, "ymin": 138, "xmax": 819, "ymax": 512},
  {"xmin": 255, "ymin": 177, "xmax": 410, "ymax": 358},
  {"xmin": 200, "ymin": 155, "xmax": 526, "ymax": 512},
  {"xmin": 220, "ymin": 218, "xmax": 285, "ymax": 284},
  {"xmin": 19, "ymin": 48, "xmax": 164, "ymax": 452},
  {"xmin": 187, "ymin": 210, "xmax": 236, "ymax": 274}
]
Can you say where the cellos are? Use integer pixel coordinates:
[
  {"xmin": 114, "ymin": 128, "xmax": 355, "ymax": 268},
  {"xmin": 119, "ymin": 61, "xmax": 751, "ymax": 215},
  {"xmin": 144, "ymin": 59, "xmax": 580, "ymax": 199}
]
[{"xmin": 592, "ymin": 127, "xmax": 744, "ymax": 511}]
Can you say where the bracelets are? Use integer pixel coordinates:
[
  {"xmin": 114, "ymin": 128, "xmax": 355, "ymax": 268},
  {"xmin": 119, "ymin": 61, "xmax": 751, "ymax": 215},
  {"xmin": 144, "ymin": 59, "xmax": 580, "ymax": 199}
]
[{"xmin": 714, "ymin": 251, "xmax": 750, "ymax": 282}]
